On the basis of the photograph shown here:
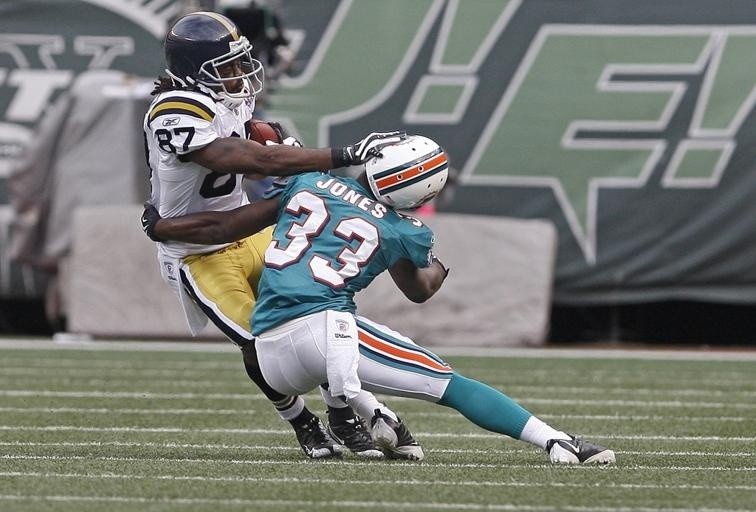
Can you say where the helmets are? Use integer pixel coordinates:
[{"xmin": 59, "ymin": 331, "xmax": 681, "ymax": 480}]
[
  {"xmin": 365, "ymin": 135, "xmax": 449, "ymax": 210},
  {"xmin": 163, "ymin": 11, "xmax": 243, "ymax": 98}
]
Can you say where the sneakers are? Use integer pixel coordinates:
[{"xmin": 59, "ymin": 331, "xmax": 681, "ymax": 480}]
[
  {"xmin": 296, "ymin": 409, "xmax": 424, "ymax": 460},
  {"xmin": 547, "ymin": 430, "xmax": 615, "ymax": 464}
]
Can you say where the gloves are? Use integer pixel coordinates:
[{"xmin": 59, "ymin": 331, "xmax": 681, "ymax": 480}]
[
  {"xmin": 266, "ymin": 121, "xmax": 303, "ymax": 147},
  {"xmin": 140, "ymin": 201, "xmax": 161, "ymax": 242},
  {"xmin": 341, "ymin": 130, "xmax": 406, "ymax": 167}
]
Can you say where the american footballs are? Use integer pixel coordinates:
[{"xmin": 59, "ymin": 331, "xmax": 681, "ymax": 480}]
[{"xmin": 248, "ymin": 119, "xmax": 280, "ymax": 149}]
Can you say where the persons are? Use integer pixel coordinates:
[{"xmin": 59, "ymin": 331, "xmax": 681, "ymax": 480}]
[
  {"xmin": 146, "ymin": 9, "xmax": 381, "ymax": 462},
  {"xmin": 139, "ymin": 131, "xmax": 619, "ymax": 466}
]
